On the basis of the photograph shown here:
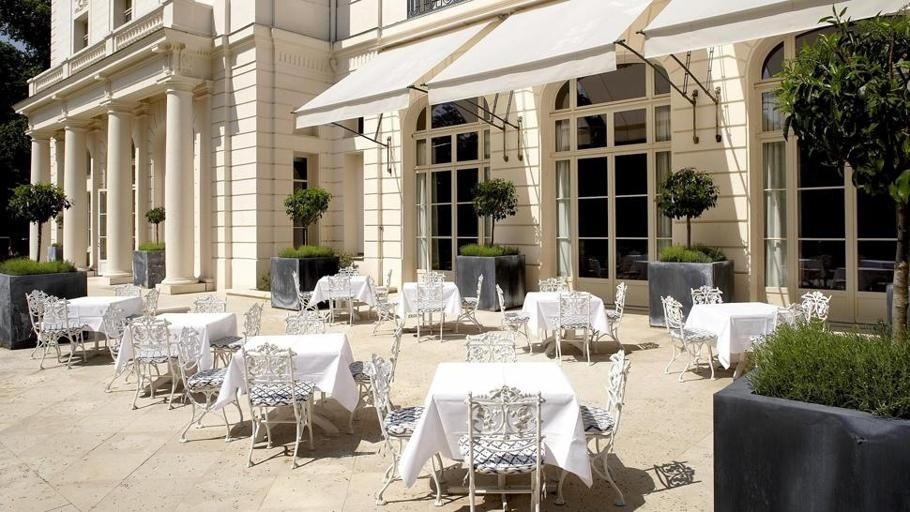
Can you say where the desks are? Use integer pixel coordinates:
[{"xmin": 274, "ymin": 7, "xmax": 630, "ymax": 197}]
[
  {"xmin": 213, "ymin": 333, "xmax": 359, "ymax": 443},
  {"xmin": 397, "ymin": 361, "xmax": 593, "ymax": 494}
]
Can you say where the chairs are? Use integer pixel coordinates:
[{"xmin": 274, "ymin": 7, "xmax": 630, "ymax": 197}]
[
  {"xmin": 173, "ymin": 326, "xmax": 246, "ymax": 443},
  {"xmin": 346, "ymin": 320, "xmax": 405, "ymax": 436},
  {"xmin": 455, "ymin": 385, "xmax": 547, "ymax": 512},
  {"xmin": 661, "ymin": 285, "xmax": 832, "ymax": 382},
  {"xmin": 463, "ymin": 331, "xmax": 517, "ymax": 363},
  {"xmin": 284, "ymin": 311, "xmax": 327, "ymax": 407},
  {"xmin": 291, "ymin": 266, "xmax": 393, "ymax": 328},
  {"xmin": 368, "ymin": 270, "xmax": 483, "ymax": 343},
  {"xmin": 552, "ymin": 349, "xmax": 632, "ymax": 505},
  {"xmin": 363, "ymin": 353, "xmax": 448, "ymax": 507},
  {"xmin": 104, "ymin": 293, "xmax": 264, "ymax": 410},
  {"xmin": 241, "ymin": 342, "xmax": 314, "ymax": 469},
  {"xmin": 496, "ymin": 277, "xmax": 628, "ymax": 367},
  {"xmin": 25, "ymin": 283, "xmax": 160, "ymax": 370}
]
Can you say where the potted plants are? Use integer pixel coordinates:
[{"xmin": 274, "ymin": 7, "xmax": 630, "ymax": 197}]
[
  {"xmin": 270, "ymin": 184, "xmax": 340, "ymax": 310},
  {"xmin": 645, "ymin": 164, "xmax": 735, "ymax": 327},
  {"xmin": 1, "ymin": 182, "xmax": 90, "ymax": 352},
  {"xmin": 710, "ymin": 3, "xmax": 909, "ymax": 509},
  {"xmin": 452, "ymin": 176, "xmax": 526, "ymax": 311},
  {"xmin": 133, "ymin": 204, "xmax": 166, "ymax": 288}
]
